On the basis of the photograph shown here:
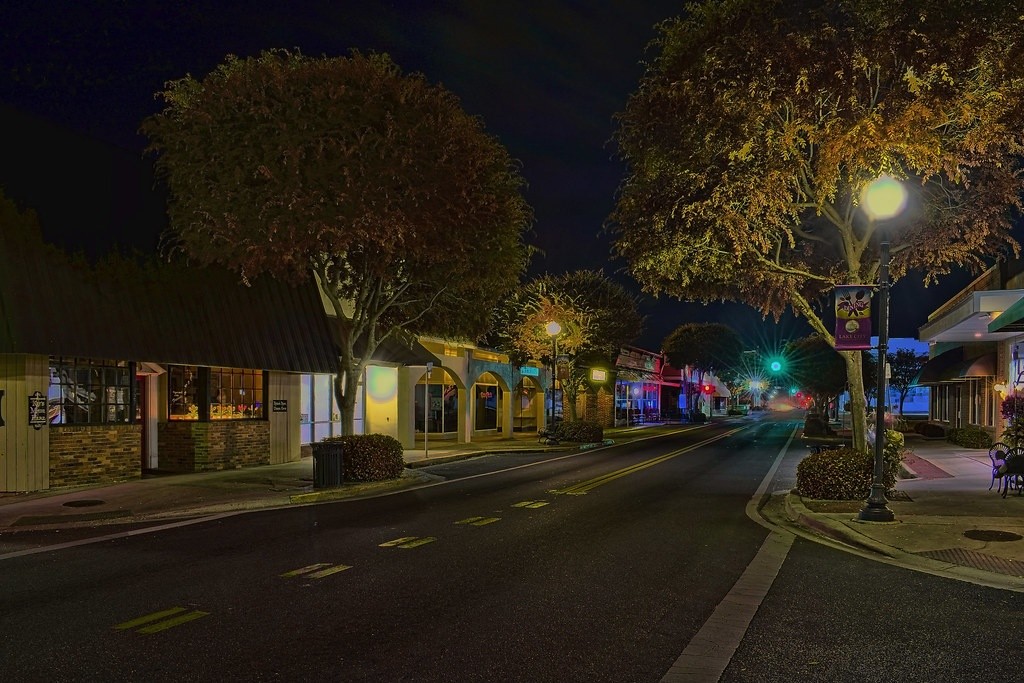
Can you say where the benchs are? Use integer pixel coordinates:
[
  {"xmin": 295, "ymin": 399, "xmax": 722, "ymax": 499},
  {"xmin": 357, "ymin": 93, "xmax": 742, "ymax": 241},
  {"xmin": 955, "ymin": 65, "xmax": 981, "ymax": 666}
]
[
  {"xmin": 538, "ymin": 424, "xmax": 559, "ymax": 446},
  {"xmin": 631, "ymin": 412, "xmax": 662, "ymax": 425}
]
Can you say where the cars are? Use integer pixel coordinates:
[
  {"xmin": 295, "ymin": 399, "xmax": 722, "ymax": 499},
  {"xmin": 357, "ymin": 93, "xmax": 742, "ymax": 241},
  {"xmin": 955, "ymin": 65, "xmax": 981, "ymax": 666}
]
[{"xmin": 729, "ymin": 405, "xmax": 748, "ymax": 416}]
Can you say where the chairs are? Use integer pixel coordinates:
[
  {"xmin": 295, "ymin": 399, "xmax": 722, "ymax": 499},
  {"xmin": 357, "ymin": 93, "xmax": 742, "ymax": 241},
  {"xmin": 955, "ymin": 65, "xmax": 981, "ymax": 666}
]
[{"xmin": 989, "ymin": 442, "xmax": 1024, "ymax": 498}]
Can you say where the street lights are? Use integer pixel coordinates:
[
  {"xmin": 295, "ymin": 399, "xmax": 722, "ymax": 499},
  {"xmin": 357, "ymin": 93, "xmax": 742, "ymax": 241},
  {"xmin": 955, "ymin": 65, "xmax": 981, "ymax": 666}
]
[
  {"xmin": 545, "ymin": 321, "xmax": 561, "ymax": 445},
  {"xmin": 860, "ymin": 168, "xmax": 909, "ymax": 522}
]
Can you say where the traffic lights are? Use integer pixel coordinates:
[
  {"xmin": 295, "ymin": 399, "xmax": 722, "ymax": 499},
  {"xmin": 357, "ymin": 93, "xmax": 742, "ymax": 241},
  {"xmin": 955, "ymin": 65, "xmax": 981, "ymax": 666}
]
[
  {"xmin": 703, "ymin": 385, "xmax": 716, "ymax": 394},
  {"xmin": 791, "ymin": 387, "xmax": 799, "ymax": 394},
  {"xmin": 771, "ymin": 360, "xmax": 783, "ymax": 373}
]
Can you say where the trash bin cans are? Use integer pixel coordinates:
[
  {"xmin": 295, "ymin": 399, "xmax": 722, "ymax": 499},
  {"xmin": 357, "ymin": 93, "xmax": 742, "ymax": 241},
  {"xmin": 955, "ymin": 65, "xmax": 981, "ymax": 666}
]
[{"xmin": 309, "ymin": 441, "xmax": 346, "ymax": 488}]
